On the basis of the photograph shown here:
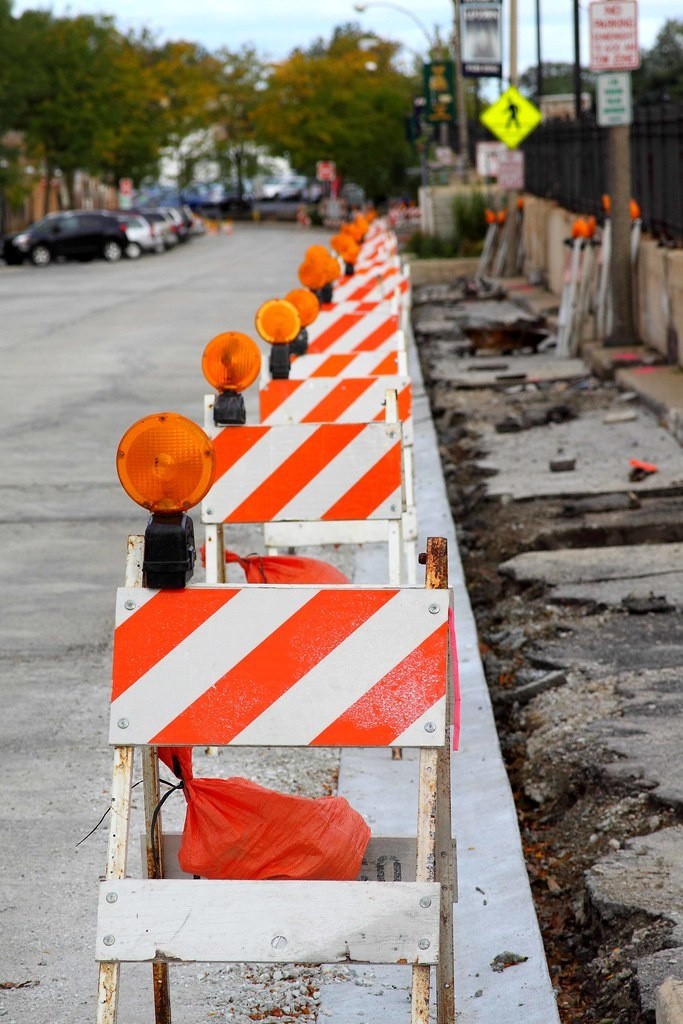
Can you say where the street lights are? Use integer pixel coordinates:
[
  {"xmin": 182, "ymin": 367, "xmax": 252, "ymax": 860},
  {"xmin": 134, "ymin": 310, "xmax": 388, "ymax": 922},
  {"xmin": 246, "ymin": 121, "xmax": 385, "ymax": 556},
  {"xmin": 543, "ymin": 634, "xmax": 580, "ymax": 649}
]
[{"xmin": 353, "ymin": 1, "xmax": 436, "ymax": 50}]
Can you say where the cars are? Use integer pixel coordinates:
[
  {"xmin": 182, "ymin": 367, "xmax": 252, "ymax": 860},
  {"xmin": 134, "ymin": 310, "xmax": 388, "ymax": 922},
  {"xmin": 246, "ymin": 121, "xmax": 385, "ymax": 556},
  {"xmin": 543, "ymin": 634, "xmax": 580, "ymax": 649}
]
[
  {"xmin": 0, "ymin": 210, "xmax": 131, "ymax": 266},
  {"xmin": 113, "ymin": 170, "xmax": 365, "ymax": 258}
]
